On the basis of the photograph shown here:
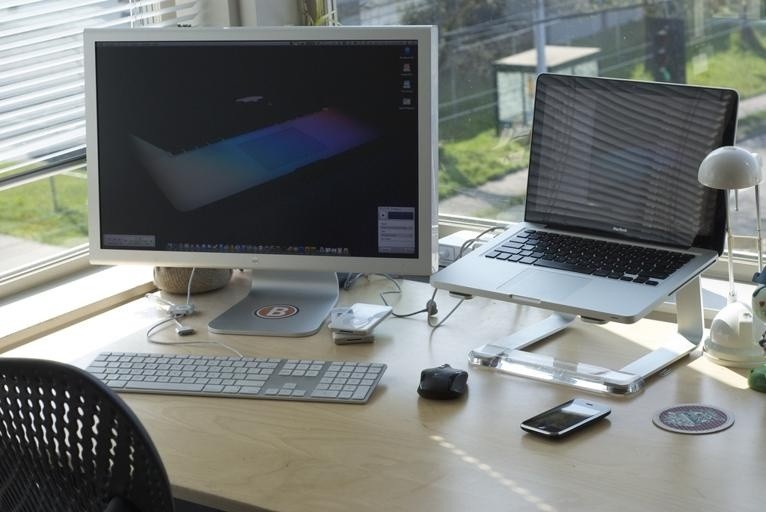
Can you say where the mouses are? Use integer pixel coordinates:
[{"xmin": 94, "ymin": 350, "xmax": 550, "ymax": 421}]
[{"xmin": 417, "ymin": 364, "xmax": 468, "ymax": 400}]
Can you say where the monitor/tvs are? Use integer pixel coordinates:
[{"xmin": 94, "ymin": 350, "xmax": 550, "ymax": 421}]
[{"xmin": 83, "ymin": 24, "xmax": 439, "ymax": 338}]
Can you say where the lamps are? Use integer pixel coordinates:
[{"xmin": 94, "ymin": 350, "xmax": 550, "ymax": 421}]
[{"xmin": 697, "ymin": 147, "xmax": 766, "ymax": 368}]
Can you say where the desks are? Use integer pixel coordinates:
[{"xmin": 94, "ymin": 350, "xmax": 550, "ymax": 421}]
[{"xmin": 1, "ymin": 274, "xmax": 766, "ymax": 512}]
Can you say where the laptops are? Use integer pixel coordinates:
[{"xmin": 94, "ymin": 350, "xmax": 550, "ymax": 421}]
[{"xmin": 429, "ymin": 73, "xmax": 738, "ymax": 324}]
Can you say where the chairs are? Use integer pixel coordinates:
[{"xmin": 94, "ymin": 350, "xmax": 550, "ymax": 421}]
[{"xmin": 0, "ymin": 357, "xmax": 176, "ymax": 512}]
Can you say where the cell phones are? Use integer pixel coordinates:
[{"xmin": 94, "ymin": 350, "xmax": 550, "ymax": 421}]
[
  {"xmin": 327, "ymin": 302, "xmax": 393, "ymax": 335},
  {"xmin": 520, "ymin": 397, "xmax": 611, "ymax": 440},
  {"xmin": 331, "ymin": 307, "xmax": 375, "ymax": 345}
]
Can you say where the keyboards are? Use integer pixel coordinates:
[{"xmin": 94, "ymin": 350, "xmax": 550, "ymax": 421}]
[{"xmin": 84, "ymin": 350, "xmax": 387, "ymax": 404}]
